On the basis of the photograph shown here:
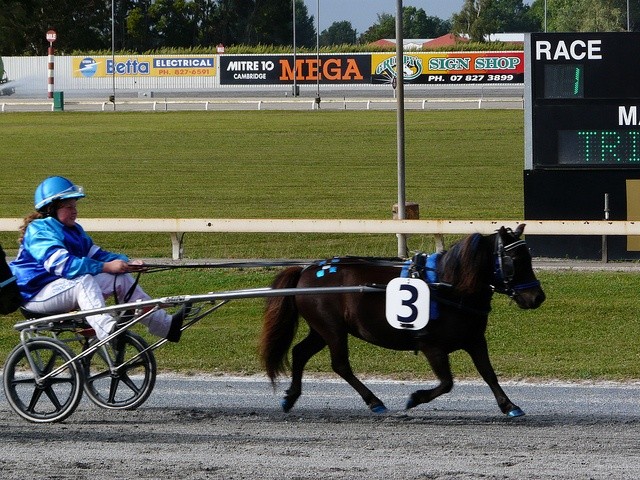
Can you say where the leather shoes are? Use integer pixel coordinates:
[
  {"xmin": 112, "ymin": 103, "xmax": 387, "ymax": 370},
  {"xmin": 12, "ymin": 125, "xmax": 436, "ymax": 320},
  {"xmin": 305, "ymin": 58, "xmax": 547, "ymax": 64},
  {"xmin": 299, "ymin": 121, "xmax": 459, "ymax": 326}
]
[
  {"xmin": 165, "ymin": 299, "xmax": 192, "ymax": 343},
  {"xmin": 109, "ymin": 306, "xmax": 135, "ymax": 352}
]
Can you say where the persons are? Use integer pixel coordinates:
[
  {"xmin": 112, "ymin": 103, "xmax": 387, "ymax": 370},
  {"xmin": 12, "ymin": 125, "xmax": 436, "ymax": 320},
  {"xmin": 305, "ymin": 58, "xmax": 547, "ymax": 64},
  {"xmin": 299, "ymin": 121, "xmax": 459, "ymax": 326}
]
[{"xmin": 7, "ymin": 175, "xmax": 193, "ymax": 343}]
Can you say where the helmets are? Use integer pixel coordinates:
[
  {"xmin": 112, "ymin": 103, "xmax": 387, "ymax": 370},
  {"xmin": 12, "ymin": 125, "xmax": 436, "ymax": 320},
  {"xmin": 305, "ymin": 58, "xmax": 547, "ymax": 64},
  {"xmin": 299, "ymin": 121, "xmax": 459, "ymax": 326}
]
[{"xmin": 34, "ymin": 175, "xmax": 86, "ymax": 211}]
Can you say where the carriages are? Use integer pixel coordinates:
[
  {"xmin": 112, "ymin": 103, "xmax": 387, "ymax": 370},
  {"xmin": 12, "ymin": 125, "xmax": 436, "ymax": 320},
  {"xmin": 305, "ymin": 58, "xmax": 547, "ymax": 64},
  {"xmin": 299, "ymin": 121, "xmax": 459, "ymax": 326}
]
[{"xmin": 2, "ymin": 223, "xmax": 543, "ymax": 422}]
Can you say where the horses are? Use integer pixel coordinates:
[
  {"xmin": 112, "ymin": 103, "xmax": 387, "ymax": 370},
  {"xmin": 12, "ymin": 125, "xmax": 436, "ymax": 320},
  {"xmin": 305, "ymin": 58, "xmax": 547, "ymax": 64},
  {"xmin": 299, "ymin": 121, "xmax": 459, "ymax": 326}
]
[{"xmin": 259, "ymin": 224, "xmax": 545, "ymax": 419}]
